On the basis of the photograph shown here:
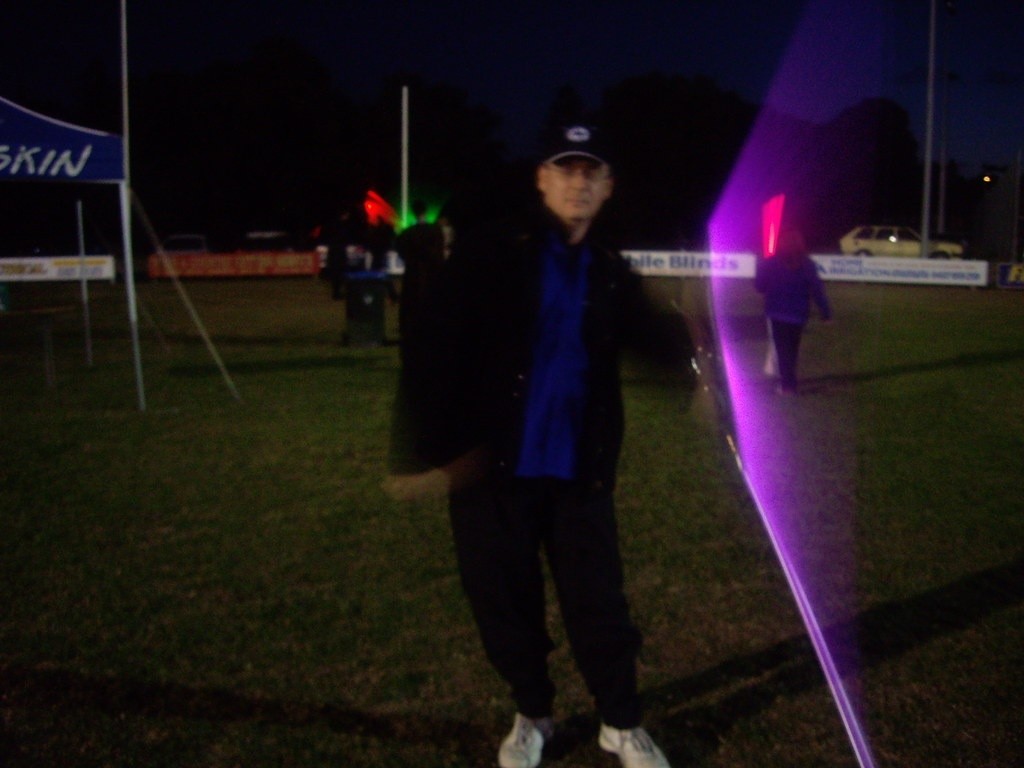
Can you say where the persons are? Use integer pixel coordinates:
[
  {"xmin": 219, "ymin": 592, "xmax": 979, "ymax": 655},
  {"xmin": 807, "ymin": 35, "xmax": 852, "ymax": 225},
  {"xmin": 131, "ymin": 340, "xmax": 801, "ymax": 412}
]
[
  {"xmin": 753, "ymin": 228, "xmax": 832, "ymax": 398},
  {"xmin": 390, "ymin": 117, "xmax": 700, "ymax": 768},
  {"xmin": 394, "ymin": 200, "xmax": 444, "ymax": 330},
  {"xmin": 319, "ymin": 206, "xmax": 370, "ymax": 295}
]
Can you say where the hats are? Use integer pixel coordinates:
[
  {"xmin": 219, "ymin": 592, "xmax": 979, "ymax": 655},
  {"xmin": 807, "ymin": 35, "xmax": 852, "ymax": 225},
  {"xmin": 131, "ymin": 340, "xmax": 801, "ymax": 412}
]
[{"xmin": 539, "ymin": 120, "xmax": 615, "ymax": 174}]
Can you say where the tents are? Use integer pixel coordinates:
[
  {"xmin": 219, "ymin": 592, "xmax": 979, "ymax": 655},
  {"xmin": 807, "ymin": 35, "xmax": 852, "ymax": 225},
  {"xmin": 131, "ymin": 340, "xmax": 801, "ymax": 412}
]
[{"xmin": 0, "ymin": 96, "xmax": 147, "ymax": 408}]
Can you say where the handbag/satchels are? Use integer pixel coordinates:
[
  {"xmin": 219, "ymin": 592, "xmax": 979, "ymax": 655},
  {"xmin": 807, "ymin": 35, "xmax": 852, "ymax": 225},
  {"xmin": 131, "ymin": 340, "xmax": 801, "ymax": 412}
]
[{"xmin": 752, "ymin": 255, "xmax": 786, "ymax": 299}]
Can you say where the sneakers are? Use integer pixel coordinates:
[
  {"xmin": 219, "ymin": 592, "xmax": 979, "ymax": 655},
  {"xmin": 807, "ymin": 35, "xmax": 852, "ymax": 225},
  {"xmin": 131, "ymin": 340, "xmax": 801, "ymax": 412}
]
[
  {"xmin": 597, "ymin": 721, "xmax": 672, "ymax": 768},
  {"xmin": 497, "ymin": 712, "xmax": 554, "ymax": 768}
]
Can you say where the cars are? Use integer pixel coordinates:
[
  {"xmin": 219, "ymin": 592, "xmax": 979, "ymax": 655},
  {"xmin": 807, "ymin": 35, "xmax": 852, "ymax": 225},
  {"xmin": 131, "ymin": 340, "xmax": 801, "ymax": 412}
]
[{"xmin": 838, "ymin": 225, "xmax": 966, "ymax": 259}]
